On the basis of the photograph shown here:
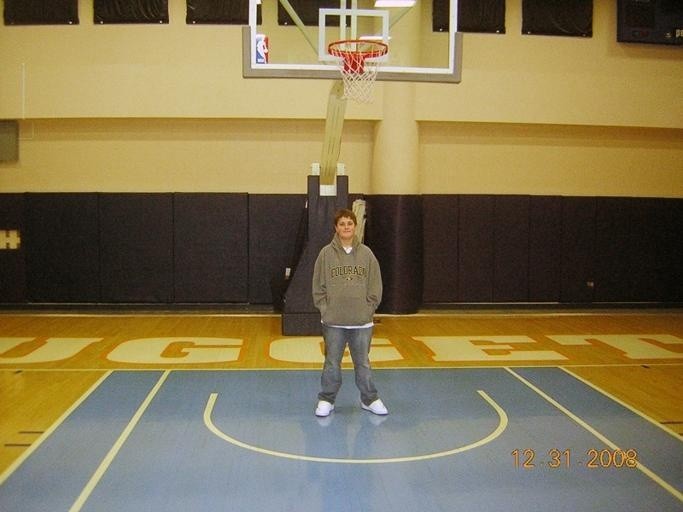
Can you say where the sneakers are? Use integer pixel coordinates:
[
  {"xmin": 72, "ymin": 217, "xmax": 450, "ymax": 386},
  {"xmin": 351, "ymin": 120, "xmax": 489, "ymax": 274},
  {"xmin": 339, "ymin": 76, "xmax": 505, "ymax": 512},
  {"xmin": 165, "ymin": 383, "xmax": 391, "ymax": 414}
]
[
  {"xmin": 316, "ymin": 400, "xmax": 334, "ymax": 416},
  {"xmin": 361, "ymin": 398, "xmax": 388, "ymax": 415}
]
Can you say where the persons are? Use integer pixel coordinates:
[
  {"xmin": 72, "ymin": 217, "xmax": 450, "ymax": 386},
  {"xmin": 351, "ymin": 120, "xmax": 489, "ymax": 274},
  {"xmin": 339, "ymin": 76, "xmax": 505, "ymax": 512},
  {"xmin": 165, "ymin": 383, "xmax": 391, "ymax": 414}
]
[{"xmin": 311, "ymin": 209, "xmax": 390, "ymax": 418}]
